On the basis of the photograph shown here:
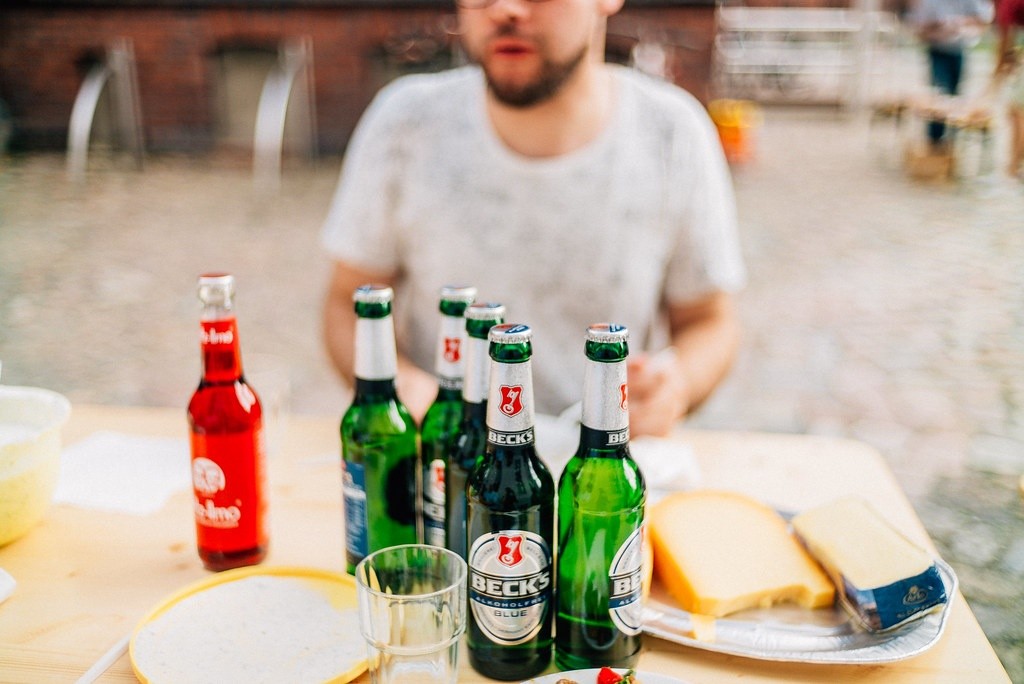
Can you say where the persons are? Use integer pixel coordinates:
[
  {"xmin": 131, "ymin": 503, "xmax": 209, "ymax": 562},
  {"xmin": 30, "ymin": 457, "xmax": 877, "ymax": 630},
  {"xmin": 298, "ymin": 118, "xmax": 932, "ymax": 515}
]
[
  {"xmin": 908, "ymin": 0, "xmax": 996, "ymax": 157},
  {"xmin": 319, "ymin": 0, "xmax": 742, "ymax": 438}
]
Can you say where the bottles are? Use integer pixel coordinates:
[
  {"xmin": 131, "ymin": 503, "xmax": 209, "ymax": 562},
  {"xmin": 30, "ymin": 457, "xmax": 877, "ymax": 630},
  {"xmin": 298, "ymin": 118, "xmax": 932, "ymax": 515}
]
[
  {"xmin": 466, "ymin": 323, "xmax": 557, "ymax": 684},
  {"xmin": 186, "ymin": 273, "xmax": 268, "ymax": 572},
  {"xmin": 554, "ymin": 320, "xmax": 645, "ymax": 672},
  {"xmin": 444, "ymin": 302, "xmax": 505, "ymax": 564},
  {"xmin": 334, "ymin": 285, "xmax": 421, "ymax": 574},
  {"xmin": 420, "ymin": 284, "xmax": 476, "ymax": 550}
]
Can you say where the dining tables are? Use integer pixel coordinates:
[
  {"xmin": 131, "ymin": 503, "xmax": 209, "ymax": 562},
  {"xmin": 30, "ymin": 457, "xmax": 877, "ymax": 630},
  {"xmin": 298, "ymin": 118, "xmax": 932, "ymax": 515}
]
[{"xmin": 0, "ymin": 405, "xmax": 1013, "ymax": 684}]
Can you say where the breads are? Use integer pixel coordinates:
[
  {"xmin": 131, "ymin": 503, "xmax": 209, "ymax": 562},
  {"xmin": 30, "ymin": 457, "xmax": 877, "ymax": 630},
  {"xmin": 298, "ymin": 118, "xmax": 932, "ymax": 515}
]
[
  {"xmin": 791, "ymin": 492, "xmax": 947, "ymax": 630},
  {"xmin": 647, "ymin": 490, "xmax": 836, "ymax": 618}
]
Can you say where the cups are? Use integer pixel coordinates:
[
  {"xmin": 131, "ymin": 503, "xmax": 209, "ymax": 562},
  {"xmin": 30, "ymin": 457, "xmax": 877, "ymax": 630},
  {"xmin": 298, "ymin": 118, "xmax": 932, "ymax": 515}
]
[{"xmin": 355, "ymin": 543, "xmax": 468, "ymax": 684}]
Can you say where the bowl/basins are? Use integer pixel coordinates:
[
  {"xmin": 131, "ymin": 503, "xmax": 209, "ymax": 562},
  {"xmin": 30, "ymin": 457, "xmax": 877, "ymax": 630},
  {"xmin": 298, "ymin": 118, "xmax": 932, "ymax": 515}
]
[{"xmin": 0, "ymin": 384, "xmax": 72, "ymax": 548}]
